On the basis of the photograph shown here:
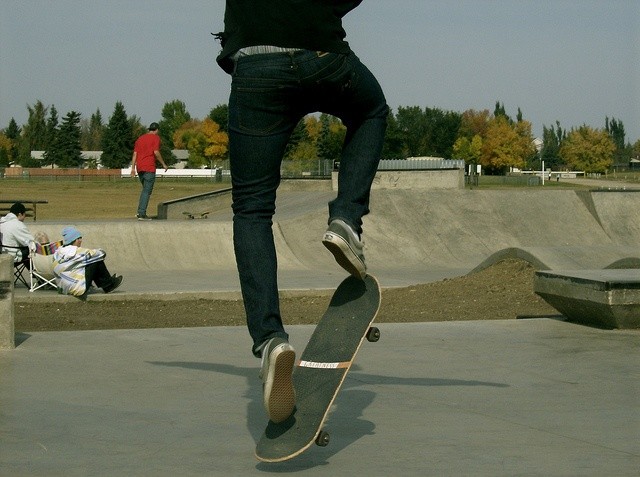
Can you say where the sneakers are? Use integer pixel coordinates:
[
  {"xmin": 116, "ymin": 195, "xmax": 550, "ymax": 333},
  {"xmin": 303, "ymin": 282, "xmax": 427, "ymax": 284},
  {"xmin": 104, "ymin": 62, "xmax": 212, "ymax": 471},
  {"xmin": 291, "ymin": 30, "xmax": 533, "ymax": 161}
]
[
  {"xmin": 258, "ymin": 337, "xmax": 297, "ymax": 424},
  {"xmin": 137, "ymin": 214, "xmax": 152, "ymax": 221},
  {"xmin": 103, "ymin": 273, "xmax": 124, "ymax": 293},
  {"xmin": 322, "ymin": 219, "xmax": 366, "ymax": 280}
]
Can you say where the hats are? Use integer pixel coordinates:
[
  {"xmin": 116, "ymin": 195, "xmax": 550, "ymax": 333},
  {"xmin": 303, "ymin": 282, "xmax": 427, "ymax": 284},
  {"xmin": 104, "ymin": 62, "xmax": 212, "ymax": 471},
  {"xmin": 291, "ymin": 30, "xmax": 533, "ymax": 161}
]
[
  {"xmin": 62, "ymin": 226, "xmax": 82, "ymax": 245},
  {"xmin": 149, "ymin": 123, "xmax": 159, "ymax": 129},
  {"xmin": 34, "ymin": 232, "xmax": 50, "ymax": 245}
]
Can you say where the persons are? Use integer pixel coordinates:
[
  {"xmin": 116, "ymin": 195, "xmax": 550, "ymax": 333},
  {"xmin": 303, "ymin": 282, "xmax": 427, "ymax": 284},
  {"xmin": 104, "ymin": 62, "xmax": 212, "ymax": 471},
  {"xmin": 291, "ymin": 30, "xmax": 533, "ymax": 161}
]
[
  {"xmin": 131, "ymin": 122, "xmax": 167, "ymax": 219},
  {"xmin": 212, "ymin": 3, "xmax": 390, "ymax": 425},
  {"xmin": 54, "ymin": 227, "xmax": 123, "ymax": 301},
  {"xmin": 0, "ymin": 202, "xmax": 37, "ymax": 277}
]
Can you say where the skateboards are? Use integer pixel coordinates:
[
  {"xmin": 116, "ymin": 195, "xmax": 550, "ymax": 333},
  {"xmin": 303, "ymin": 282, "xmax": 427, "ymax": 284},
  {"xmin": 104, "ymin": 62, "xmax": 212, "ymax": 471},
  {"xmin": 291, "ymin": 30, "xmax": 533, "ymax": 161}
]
[
  {"xmin": 182, "ymin": 211, "xmax": 211, "ymax": 219},
  {"xmin": 253, "ymin": 272, "xmax": 381, "ymax": 462}
]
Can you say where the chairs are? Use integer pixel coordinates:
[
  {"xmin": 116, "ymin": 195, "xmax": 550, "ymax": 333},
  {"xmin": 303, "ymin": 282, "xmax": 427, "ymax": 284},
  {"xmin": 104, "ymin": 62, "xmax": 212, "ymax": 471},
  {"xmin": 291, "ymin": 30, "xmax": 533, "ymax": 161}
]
[
  {"xmin": 0, "ymin": 244, "xmax": 31, "ymax": 290},
  {"xmin": 28, "ymin": 240, "xmax": 63, "ymax": 292}
]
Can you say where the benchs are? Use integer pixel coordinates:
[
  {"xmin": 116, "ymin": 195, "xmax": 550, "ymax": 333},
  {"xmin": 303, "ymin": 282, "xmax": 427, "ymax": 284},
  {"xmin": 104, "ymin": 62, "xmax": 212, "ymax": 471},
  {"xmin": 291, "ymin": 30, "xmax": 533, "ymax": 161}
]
[{"xmin": 0, "ymin": 199, "xmax": 48, "ymax": 221}]
